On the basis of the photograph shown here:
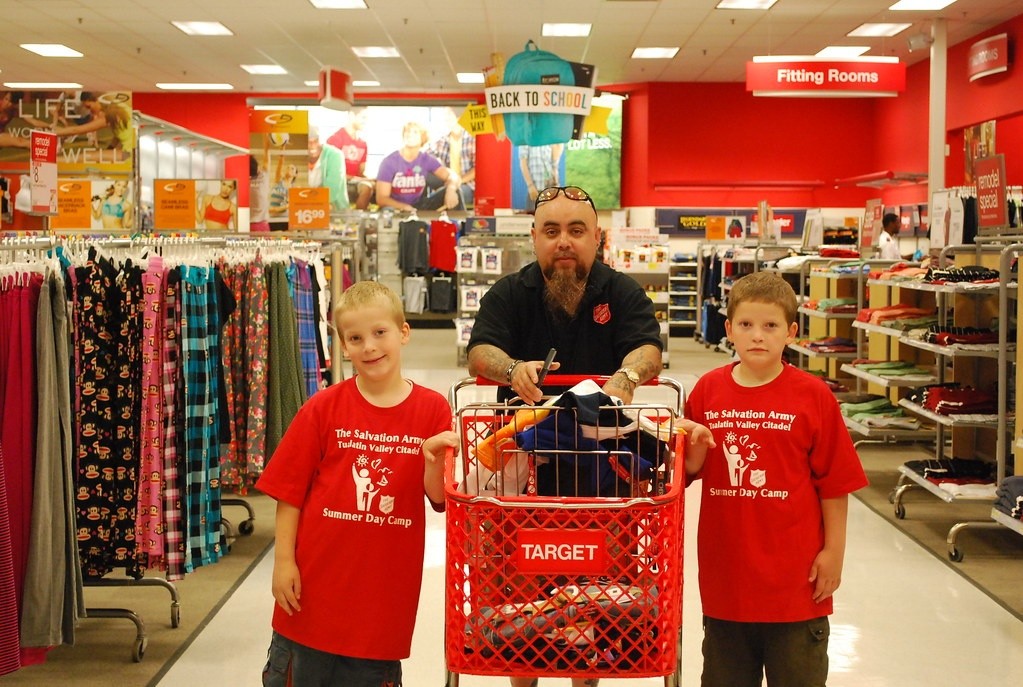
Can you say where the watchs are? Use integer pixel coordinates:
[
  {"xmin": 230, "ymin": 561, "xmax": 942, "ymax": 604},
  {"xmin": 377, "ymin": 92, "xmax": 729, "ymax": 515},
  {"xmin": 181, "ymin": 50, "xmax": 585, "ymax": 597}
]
[{"xmin": 617, "ymin": 368, "xmax": 640, "ymax": 388}]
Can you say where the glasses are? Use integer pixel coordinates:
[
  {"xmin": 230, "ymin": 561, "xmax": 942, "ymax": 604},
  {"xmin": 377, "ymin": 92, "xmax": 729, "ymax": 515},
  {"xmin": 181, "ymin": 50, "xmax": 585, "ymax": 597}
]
[{"xmin": 535, "ymin": 185, "xmax": 597, "ymax": 218}]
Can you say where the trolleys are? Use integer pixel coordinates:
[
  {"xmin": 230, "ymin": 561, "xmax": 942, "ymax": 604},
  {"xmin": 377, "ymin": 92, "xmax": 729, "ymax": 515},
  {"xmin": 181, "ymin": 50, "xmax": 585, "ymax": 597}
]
[{"xmin": 441, "ymin": 372, "xmax": 691, "ymax": 687}]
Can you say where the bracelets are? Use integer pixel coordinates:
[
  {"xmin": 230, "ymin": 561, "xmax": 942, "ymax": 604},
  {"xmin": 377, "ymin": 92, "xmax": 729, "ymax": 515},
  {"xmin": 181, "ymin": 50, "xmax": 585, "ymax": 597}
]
[{"xmin": 506, "ymin": 360, "xmax": 523, "ymax": 389}]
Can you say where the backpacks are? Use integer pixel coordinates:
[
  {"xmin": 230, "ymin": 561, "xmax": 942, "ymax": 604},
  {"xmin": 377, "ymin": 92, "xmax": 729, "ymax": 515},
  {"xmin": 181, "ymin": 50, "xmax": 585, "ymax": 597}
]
[{"xmin": 501, "ymin": 39, "xmax": 574, "ymax": 146}]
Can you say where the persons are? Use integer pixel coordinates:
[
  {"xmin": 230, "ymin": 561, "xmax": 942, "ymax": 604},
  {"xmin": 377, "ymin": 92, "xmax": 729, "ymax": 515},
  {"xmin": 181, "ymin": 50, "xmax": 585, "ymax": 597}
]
[
  {"xmin": 519, "ymin": 142, "xmax": 564, "ymax": 210},
  {"xmin": 270, "ymin": 141, "xmax": 298, "ymax": 216},
  {"xmin": 376, "ymin": 121, "xmax": 466, "ymax": 212},
  {"xmin": 327, "ymin": 121, "xmax": 378, "ymax": 212},
  {"xmin": 308, "ymin": 126, "xmax": 350, "ymax": 212},
  {"xmin": 425, "ymin": 126, "xmax": 476, "ymax": 204},
  {"xmin": 195, "ymin": 180, "xmax": 236, "ymax": 230},
  {"xmin": 250, "ymin": 131, "xmax": 274, "ymax": 232},
  {"xmin": 668, "ymin": 273, "xmax": 870, "ymax": 687},
  {"xmin": 253, "ymin": 281, "xmax": 459, "ymax": 687},
  {"xmin": 466, "ymin": 194, "xmax": 662, "ymax": 687},
  {"xmin": 91, "ymin": 180, "xmax": 132, "ymax": 229},
  {"xmin": 878, "ymin": 213, "xmax": 902, "ymax": 260},
  {"xmin": 0, "ymin": 91, "xmax": 132, "ymax": 162}
]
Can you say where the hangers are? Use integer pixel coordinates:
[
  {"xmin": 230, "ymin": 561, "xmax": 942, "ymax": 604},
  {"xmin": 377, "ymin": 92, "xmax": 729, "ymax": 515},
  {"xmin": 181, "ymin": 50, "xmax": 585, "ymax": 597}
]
[
  {"xmin": 412, "ymin": 273, "xmax": 418, "ymax": 278},
  {"xmin": 438, "ymin": 211, "xmax": 451, "ymax": 224},
  {"xmin": 57, "ymin": 236, "xmax": 203, "ymax": 266},
  {"xmin": 0, "ymin": 235, "xmax": 57, "ymax": 290},
  {"xmin": 204, "ymin": 237, "xmax": 323, "ymax": 266},
  {"xmin": 323, "ymin": 244, "xmax": 353, "ymax": 263},
  {"xmin": 432, "ymin": 273, "xmax": 451, "ymax": 283},
  {"xmin": 958, "ymin": 186, "xmax": 1023, "ymax": 202},
  {"xmin": 403, "ymin": 211, "xmax": 419, "ymax": 222}
]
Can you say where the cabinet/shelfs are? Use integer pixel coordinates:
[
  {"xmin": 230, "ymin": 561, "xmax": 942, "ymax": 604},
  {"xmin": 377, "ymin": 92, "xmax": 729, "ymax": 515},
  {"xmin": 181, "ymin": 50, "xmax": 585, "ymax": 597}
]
[
  {"xmin": 754, "ymin": 241, "xmax": 1023, "ymax": 561},
  {"xmin": 670, "ymin": 262, "xmax": 697, "ymax": 327},
  {"xmin": 612, "ymin": 247, "xmax": 669, "ymax": 351}
]
